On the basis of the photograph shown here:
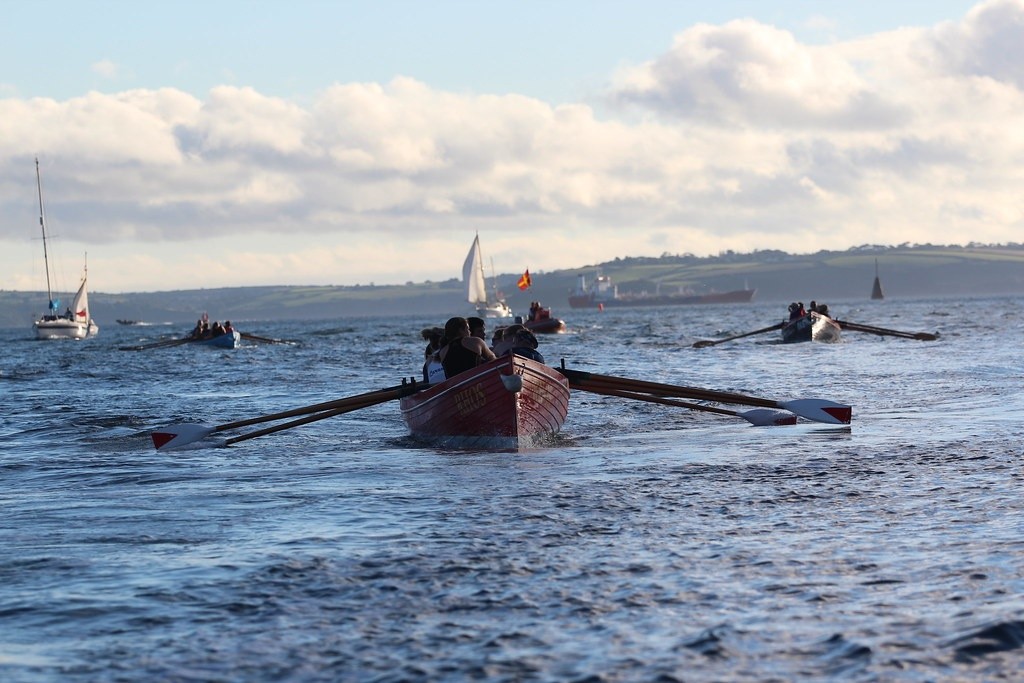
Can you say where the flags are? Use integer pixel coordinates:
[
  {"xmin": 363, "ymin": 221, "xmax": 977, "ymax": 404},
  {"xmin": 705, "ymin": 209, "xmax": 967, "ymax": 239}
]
[{"xmin": 517, "ymin": 270, "xmax": 531, "ymax": 291}]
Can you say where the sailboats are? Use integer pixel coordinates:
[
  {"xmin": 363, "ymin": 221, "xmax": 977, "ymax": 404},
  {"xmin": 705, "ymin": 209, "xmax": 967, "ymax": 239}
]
[
  {"xmin": 463, "ymin": 230, "xmax": 507, "ymax": 317},
  {"xmin": 71, "ymin": 251, "xmax": 98, "ymax": 335},
  {"xmin": 31, "ymin": 157, "xmax": 88, "ymax": 340}
]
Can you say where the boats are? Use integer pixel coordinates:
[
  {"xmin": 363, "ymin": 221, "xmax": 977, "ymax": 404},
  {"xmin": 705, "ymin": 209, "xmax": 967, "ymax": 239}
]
[
  {"xmin": 490, "ymin": 256, "xmax": 513, "ymax": 318},
  {"xmin": 782, "ymin": 311, "xmax": 842, "ymax": 343},
  {"xmin": 400, "ymin": 352, "xmax": 571, "ymax": 453},
  {"xmin": 116, "ymin": 320, "xmax": 137, "ymax": 325},
  {"xmin": 195, "ymin": 331, "xmax": 240, "ymax": 350},
  {"xmin": 568, "ymin": 274, "xmax": 755, "ymax": 308},
  {"xmin": 523, "ymin": 317, "xmax": 565, "ymax": 334}
]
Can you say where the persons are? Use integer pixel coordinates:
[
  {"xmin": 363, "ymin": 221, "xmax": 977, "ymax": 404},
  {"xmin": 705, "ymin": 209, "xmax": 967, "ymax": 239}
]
[
  {"xmin": 224, "ymin": 321, "xmax": 233, "ymax": 333},
  {"xmin": 212, "ymin": 321, "xmax": 220, "ymax": 337},
  {"xmin": 421, "ymin": 328, "xmax": 446, "ymax": 385},
  {"xmin": 789, "ymin": 300, "xmax": 806, "ymax": 321},
  {"xmin": 488, "ymin": 330, "xmax": 507, "ymax": 353},
  {"xmin": 493, "ymin": 325, "xmax": 527, "ymax": 361},
  {"xmin": 466, "ymin": 318, "xmax": 486, "ymax": 362},
  {"xmin": 440, "ymin": 317, "xmax": 497, "ymax": 380},
  {"xmin": 202, "ymin": 323, "xmax": 211, "ymax": 337},
  {"xmin": 809, "ymin": 300, "xmax": 829, "ymax": 319},
  {"xmin": 529, "ymin": 301, "xmax": 535, "ymax": 321},
  {"xmin": 535, "ymin": 302, "xmax": 540, "ymax": 311},
  {"xmin": 201, "ymin": 311, "xmax": 209, "ymax": 327},
  {"xmin": 64, "ymin": 307, "xmax": 73, "ymax": 322},
  {"xmin": 502, "ymin": 329, "xmax": 545, "ymax": 364},
  {"xmin": 195, "ymin": 320, "xmax": 204, "ymax": 336}
]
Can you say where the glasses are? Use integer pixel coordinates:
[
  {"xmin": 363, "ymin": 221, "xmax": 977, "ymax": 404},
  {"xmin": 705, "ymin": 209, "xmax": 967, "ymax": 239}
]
[{"xmin": 492, "ymin": 337, "xmax": 501, "ymax": 343}]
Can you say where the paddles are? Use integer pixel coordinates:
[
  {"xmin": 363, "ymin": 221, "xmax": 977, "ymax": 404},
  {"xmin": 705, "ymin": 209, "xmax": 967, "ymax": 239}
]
[
  {"xmin": 833, "ymin": 318, "xmax": 936, "ymax": 341},
  {"xmin": 240, "ymin": 332, "xmax": 280, "ymax": 344},
  {"xmin": 692, "ymin": 322, "xmax": 786, "ymax": 349},
  {"xmin": 553, "ymin": 366, "xmax": 853, "ymax": 427},
  {"xmin": 149, "ymin": 380, "xmax": 441, "ymax": 452},
  {"xmin": 118, "ymin": 337, "xmax": 194, "ymax": 351},
  {"xmin": 218, "ymin": 381, "xmax": 425, "ymax": 447}
]
